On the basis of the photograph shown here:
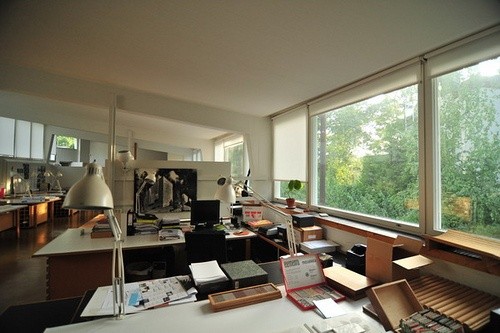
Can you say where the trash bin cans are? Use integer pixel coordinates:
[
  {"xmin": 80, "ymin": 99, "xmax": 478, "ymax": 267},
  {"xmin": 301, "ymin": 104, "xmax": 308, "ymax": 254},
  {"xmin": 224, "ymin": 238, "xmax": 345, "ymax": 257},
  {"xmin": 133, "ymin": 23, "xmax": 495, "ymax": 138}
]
[{"xmin": 345, "ymin": 244, "xmax": 367, "ymax": 275}]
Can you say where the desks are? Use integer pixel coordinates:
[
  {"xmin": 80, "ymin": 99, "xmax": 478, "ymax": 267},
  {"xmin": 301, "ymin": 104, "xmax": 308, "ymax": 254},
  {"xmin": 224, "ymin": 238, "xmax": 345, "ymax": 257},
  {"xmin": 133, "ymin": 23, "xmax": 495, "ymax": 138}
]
[
  {"xmin": 32, "ymin": 213, "xmax": 257, "ymax": 300},
  {"xmin": 0, "ymin": 189, "xmax": 82, "ymax": 235},
  {"xmin": 0, "ymin": 262, "xmax": 386, "ymax": 333}
]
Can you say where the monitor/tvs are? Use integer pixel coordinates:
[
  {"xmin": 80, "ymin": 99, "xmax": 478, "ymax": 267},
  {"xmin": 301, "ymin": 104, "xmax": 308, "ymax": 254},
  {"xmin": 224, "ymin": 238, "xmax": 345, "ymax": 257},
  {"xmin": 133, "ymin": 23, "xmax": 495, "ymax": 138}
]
[{"xmin": 191, "ymin": 200, "xmax": 220, "ymax": 232}]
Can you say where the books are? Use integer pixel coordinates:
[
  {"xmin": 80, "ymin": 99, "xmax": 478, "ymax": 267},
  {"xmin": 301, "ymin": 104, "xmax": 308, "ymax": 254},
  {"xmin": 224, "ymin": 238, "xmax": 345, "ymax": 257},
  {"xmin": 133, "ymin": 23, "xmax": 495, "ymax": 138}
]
[{"xmin": 90, "ymin": 219, "xmax": 113, "ymax": 238}]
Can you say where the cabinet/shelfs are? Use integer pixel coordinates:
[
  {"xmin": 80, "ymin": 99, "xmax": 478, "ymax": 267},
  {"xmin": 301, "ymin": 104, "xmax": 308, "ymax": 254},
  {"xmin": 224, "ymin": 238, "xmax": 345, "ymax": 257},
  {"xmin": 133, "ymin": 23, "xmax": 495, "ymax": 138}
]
[{"xmin": 251, "ymin": 237, "xmax": 289, "ymax": 262}]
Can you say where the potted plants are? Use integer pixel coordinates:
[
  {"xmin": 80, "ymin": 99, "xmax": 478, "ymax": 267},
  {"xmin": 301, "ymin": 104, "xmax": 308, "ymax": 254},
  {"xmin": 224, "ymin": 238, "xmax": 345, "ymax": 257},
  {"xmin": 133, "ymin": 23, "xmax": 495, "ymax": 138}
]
[{"xmin": 284, "ymin": 180, "xmax": 301, "ymax": 209}]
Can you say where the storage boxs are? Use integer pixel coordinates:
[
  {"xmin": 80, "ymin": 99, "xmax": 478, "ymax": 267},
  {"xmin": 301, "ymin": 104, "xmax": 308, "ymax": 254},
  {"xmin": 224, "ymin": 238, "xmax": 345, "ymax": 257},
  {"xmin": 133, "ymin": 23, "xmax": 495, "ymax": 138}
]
[
  {"xmin": 322, "ymin": 267, "xmax": 376, "ymax": 301},
  {"xmin": 366, "ymin": 235, "xmax": 433, "ymax": 282},
  {"xmin": 197, "ymin": 259, "xmax": 268, "ymax": 301},
  {"xmin": 293, "ymin": 213, "xmax": 340, "ymax": 255}
]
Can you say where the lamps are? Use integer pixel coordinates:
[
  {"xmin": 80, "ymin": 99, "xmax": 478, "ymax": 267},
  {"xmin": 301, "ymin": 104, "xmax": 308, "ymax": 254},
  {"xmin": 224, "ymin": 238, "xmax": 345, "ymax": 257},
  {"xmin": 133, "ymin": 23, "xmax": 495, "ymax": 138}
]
[
  {"xmin": 9, "ymin": 171, "xmax": 62, "ymax": 200},
  {"xmin": 136, "ymin": 172, "xmax": 156, "ymax": 215},
  {"xmin": 215, "ymin": 177, "xmax": 297, "ymax": 257},
  {"xmin": 61, "ymin": 159, "xmax": 125, "ymax": 319}
]
